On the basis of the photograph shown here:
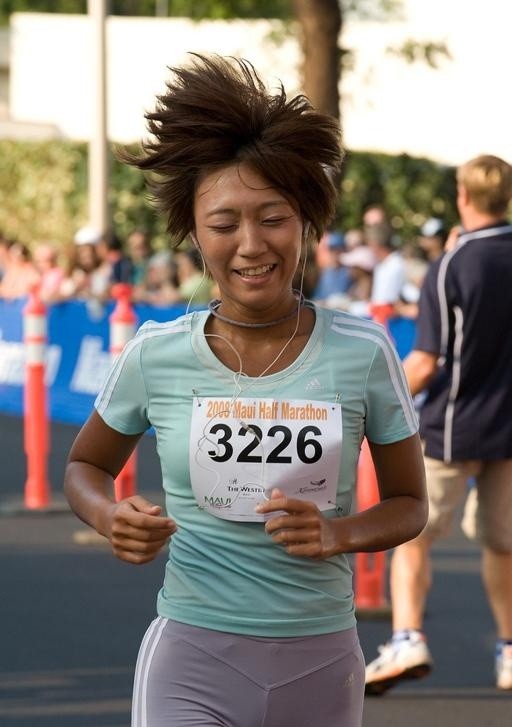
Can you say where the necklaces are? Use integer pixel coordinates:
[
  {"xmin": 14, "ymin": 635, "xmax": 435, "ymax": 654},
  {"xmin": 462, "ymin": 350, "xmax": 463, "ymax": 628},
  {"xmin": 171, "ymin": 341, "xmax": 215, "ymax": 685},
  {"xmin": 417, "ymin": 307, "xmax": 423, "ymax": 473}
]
[{"xmin": 205, "ymin": 286, "xmax": 310, "ymax": 332}]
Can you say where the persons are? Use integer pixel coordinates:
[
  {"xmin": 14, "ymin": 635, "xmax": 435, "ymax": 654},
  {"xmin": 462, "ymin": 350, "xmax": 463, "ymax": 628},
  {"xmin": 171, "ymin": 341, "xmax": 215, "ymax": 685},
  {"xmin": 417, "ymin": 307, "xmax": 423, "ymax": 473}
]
[
  {"xmin": 63, "ymin": 46, "xmax": 428, "ymax": 726},
  {"xmin": 366, "ymin": 150, "xmax": 512, "ymax": 706},
  {"xmin": 2, "ymin": 232, "xmax": 222, "ymax": 306},
  {"xmin": 308, "ymin": 205, "xmax": 465, "ymax": 322}
]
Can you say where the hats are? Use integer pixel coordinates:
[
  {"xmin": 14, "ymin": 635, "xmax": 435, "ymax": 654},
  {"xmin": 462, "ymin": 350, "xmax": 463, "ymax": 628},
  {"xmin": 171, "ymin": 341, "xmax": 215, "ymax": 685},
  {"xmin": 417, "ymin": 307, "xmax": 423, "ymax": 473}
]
[{"xmin": 325, "ymin": 216, "xmax": 445, "ymax": 272}]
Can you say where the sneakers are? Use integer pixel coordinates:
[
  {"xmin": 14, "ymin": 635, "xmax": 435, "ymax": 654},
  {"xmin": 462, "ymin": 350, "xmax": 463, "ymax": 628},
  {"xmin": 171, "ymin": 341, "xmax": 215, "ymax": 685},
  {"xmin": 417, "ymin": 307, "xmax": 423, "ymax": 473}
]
[
  {"xmin": 365, "ymin": 639, "xmax": 433, "ymax": 697},
  {"xmin": 495, "ymin": 644, "xmax": 512, "ymax": 691}
]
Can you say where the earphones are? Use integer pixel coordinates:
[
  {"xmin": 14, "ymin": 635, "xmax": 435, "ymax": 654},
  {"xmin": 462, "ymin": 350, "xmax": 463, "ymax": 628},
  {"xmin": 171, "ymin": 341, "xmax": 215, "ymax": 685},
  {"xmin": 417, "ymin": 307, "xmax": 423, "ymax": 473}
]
[
  {"xmin": 187, "ymin": 230, "xmax": 201, "ymax": 251},
  {"xmin": 303, "ymin": 220, "xmax": 313, "ymax": 239}
]
